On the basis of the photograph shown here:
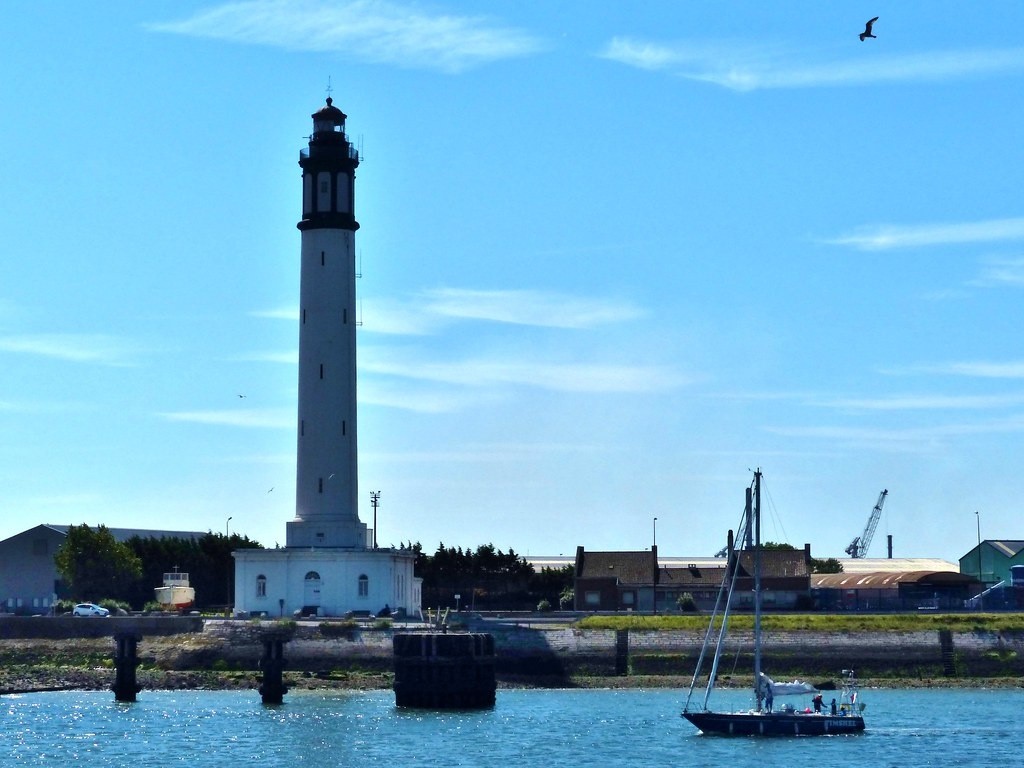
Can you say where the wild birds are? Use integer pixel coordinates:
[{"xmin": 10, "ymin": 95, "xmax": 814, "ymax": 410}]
[{"xmin": 858, "ymin": 16, "xmax": 881, "ymax": 42}]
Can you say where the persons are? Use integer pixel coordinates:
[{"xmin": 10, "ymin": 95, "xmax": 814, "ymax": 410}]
[
  {"xmin": 830, "ymin": 699, "xmax": 836, "ymax": 715},
  {"xmin": 765, "ymin": 683, "xmax": 773, "ymax": 715},
  {"xmin": 812, "ymin": 694, "xmax": 827, "ymax": 714}
]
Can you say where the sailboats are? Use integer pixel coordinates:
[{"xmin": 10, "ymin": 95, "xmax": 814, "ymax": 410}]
[{"xmin": 682, "ymin": 468, "xmax": 868, "ymax": 738}]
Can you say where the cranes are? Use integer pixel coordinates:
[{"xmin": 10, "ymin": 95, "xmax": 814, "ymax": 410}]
[{"xmin": 845, "ymin": 488, "xmax": 889, "ymax": 558}]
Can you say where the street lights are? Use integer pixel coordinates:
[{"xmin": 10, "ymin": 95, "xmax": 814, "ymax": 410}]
[
  {"xmin": 975, "ymin": 511, "xmax": 983, "ymax": 612},
  {"xmin": 652, "ymin": 517, "xmax": 658, "ymax": 544},
  {"xmin": 225, "ymin": 517, "xmax": 233, "ymax": 549},
  {"xmin": 369, "ymin": 490, "xmax": 382, "ymax": 552}
]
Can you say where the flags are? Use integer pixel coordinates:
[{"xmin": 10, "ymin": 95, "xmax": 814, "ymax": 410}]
[{"xmin": 850, "ymin": 693, "xmax": 857, "ymax": 703}]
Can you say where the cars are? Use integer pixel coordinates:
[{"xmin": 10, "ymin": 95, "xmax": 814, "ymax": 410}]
[{"xmin": 73, "ymin": 603, "xmax": 110, "ymax": 616}]
